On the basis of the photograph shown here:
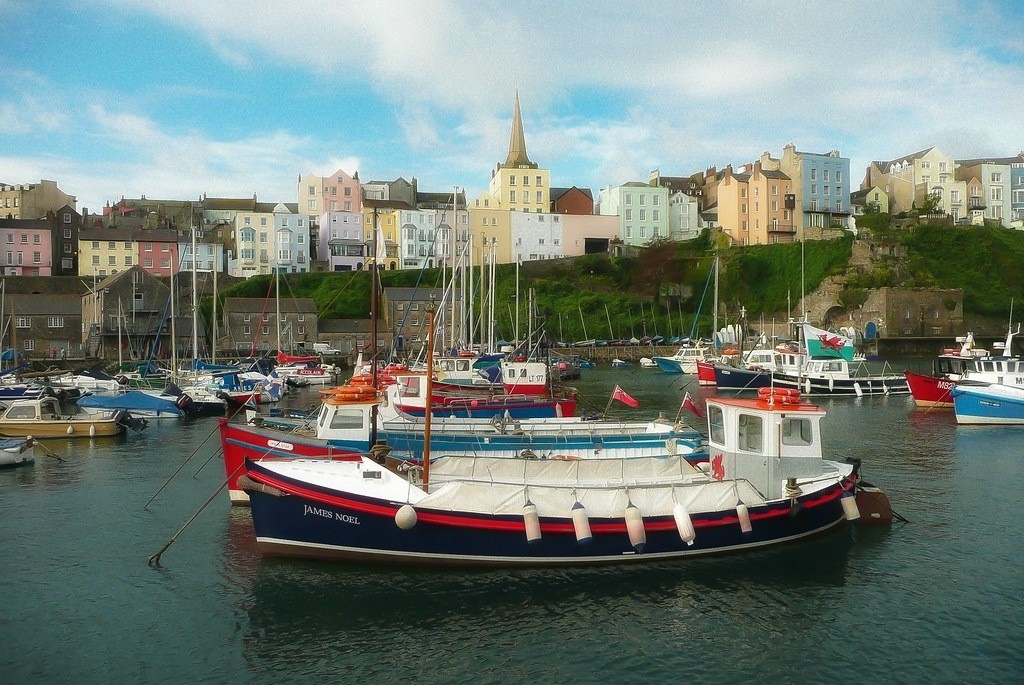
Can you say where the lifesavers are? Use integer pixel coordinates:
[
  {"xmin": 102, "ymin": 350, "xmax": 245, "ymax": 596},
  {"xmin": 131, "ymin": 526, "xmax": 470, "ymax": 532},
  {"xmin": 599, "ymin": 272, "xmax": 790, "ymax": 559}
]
[
  {"xmin": 350, "ymin": 373, "xmax": 373, "ymax": 385},
  {"xmin": 757, "ymin": 386, "xmax": 799, "ymax": 404},
  {"xmin": 335, "ymin": 384, "xmax": 376, "ymax": 399},
  {"xmin": 724, "ymin": 348, "xmax": 740, "ymax": 354},
  {"xmin": 385, "ymin": 365, "xmax": 405, "ymax": 369}
]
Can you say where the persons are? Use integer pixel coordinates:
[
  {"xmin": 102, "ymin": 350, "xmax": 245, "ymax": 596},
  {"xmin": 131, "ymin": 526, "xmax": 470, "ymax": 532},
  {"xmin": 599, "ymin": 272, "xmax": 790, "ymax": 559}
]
[
  {"xmin": 44, "ymin": 375, "xmax": 50, "ymax": 385},
  {"xmin": 50, "ymin": 346, "xmax": 59, "ymax": 359},
  {"xmin": 60, "ymin": 347, "xmax": 65, "ymax": 360}
]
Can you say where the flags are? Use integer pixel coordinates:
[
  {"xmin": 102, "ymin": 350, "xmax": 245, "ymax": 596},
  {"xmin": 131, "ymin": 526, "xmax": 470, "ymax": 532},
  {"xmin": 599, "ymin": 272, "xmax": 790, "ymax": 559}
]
[
  {"xmin": 801, "ymin": 323, "xmax": 855, "ymax": 362},
  {"xmin": 611, "ymin": 382, "xmax": 639, "ymax": 408},
  {"xmin": 682, "ymin": 391, "xmax": 703, "ymax": 417}
]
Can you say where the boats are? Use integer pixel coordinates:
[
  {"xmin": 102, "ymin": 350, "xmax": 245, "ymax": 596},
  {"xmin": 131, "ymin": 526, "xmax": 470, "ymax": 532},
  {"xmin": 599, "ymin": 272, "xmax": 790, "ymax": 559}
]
[{"xmin": 0, "ymin": 186, "xmax": 1023, "ymax": 567}]
